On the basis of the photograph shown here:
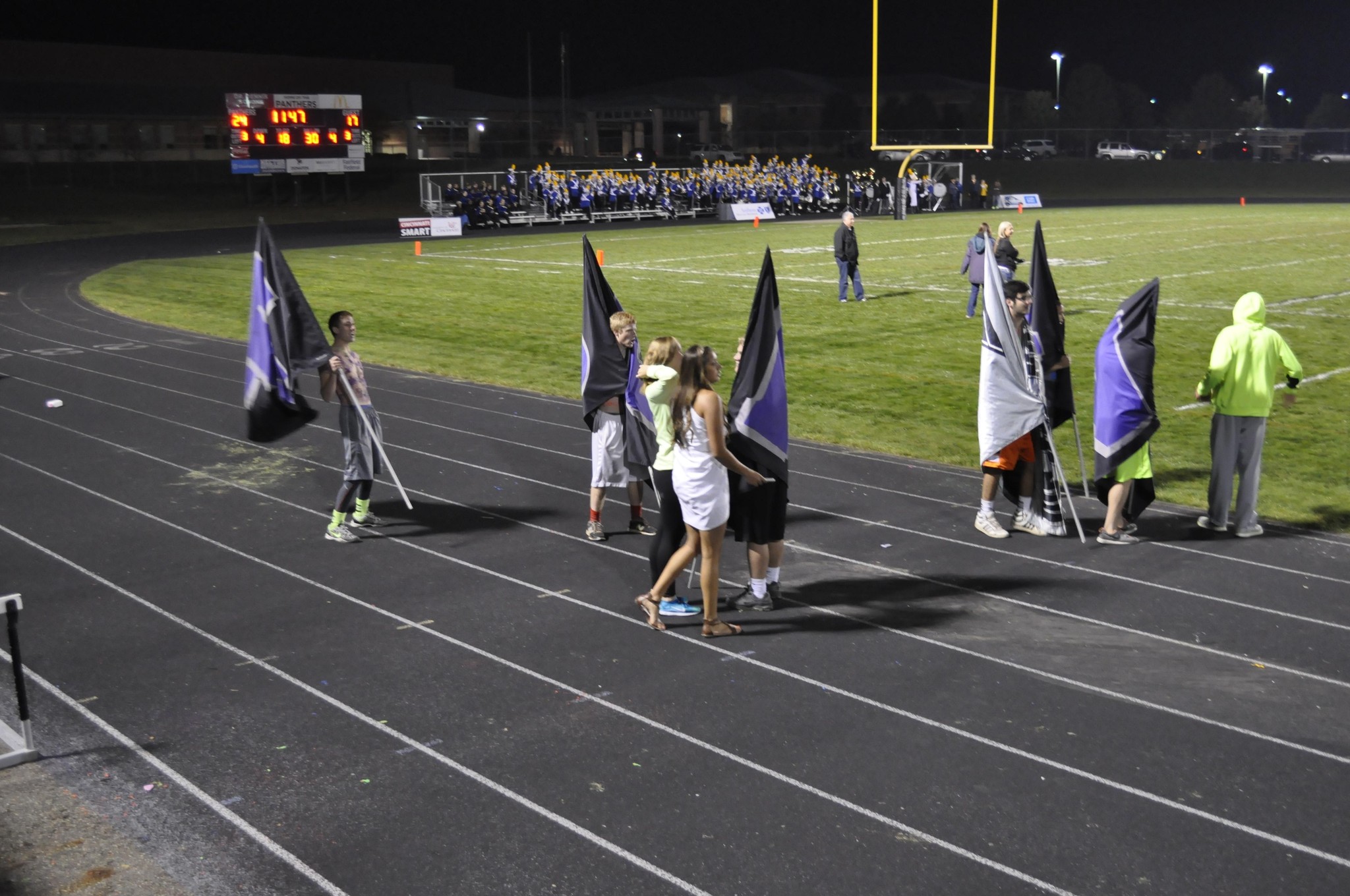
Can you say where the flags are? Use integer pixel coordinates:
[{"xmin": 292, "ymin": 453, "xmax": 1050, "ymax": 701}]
[
  {"xmin": 723, "ymin": 248, "xmax": 791, "ymax": 544},
  {"xmin": 977, "ymin": 230, "xmax": 1049, "ymax": 512},
  {"xmin": 577, "ymin": 235, "xmax": 656, "ymax": 481},
  {"xmin": 243, "ymin": 218, "xmax": 333, "ymax": 444},
  {"xmin": 1033, "ymin": 225, "xmax": 1075, "ymax": 431},
  {"xmin": 1092, "ymin": 276, "xmax": 1159, "ymax": 523}
]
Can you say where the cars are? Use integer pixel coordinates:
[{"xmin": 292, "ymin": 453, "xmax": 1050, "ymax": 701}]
[
  {"xmin": 976, "ymin": 141, "xmax": 1038, "ymax": 162},
  {"xmin": 623, "ymin": 147, "xmax": 652, "ymax": 162},
  {"xmin": 1307, "ymin": 147, "xmax": 1350, "ymax": 163},
  {"xmin": 876, "ymin": 141, "xmax": 951, "ymax": 163},
  {"xmin": 1138, "ymin": 144, "xmax": 1166, "ymax": 160}
]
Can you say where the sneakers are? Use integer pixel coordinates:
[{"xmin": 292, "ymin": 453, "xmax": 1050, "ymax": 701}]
[
  {"xmin": 974, "ymin": 511, "xmax": 1009, "ymax": 538},
  {"xmin": 628, "ymin": 516, "xmax": 656, "ymax": 536},
  {"xmin": 324, "ymin": 523, "xmax": 360, "ymax": 542},
  {"xmin": 1197, "ymin": 516, "xmax": 1227, "ymax": 531},
  {"xmin": 1096, "ymin": 529, "xmax": 1140, "ymax": 545},
  {"xmin": 586, "ymin": 520, "xmax": 605, "ymax": 541},
  {"xmin": 658, "ymin": 597, "xmax": 700, "ymax": 615},
  {"xmin": 726, "ymin": 585, "xmax": 774, "ymax": 611},
  {"xmin": 768, "ymin": 582, "xmax": 784, "ymax": 603},
  {"xmin": 1235, "ymin": 524, "xmax": 1264, "ymax": 537},
  {"xmin": 1010, "ymin": 509, "xmax": 1047, "ymax": 535},
  {"xmin": 1118, "ymin": 517, "xmax": 1137, "ymax": 534},
  {"xmin": 350, "ymin": 510, "xmax": 384, "ymax": 526}
]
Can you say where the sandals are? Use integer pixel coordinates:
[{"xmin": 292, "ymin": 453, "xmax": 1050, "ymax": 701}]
[
  {"xmin": 701, "ymin": 616, "xmax": 742, "ymax": 637},
  {"xmin": 636, "ymin": 592, "xmax": 665, "ymax": 630}
]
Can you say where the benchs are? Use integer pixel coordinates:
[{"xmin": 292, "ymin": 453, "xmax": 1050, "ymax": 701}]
[{"xmin": 421, "ymin": 194, "xmax": 721, "ymax": 228}]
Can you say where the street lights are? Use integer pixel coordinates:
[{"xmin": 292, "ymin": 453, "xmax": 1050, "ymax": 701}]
[
  {"xmin": 1050, "ymin": 53, "xmax": 1066, "ymax": 147},
  {"xmin": 1258, "ymin": 65, "xmax": 1274, "ymax": 126}
]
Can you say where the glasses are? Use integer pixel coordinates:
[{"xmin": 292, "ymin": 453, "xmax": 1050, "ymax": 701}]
[{"xmin": 1017, "ymin": 295, "xmax": 1032, "ymax": 302}]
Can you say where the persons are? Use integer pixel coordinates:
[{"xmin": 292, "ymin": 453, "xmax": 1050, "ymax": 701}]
[
  {"xmin": 974, "ymin": 282, "xmax": 1071, "ymax": 538},
  {"xmin": 994, "ymin": 221, "xmax": 1026, "ymax": 283},
  {"xmin": 834, "ymin": 211, "xmax": 868, "ymax": 303},
  {"xmin": 949, "ymin": 177, "xmax": 963, "ymax": 210},
  {"xmin": 966, "ymin": 174, "xmax": 1000, "ymax": 210},
  {"xmin": 506, "ymin": 168, "xmax": 518, "ymax": 188},
  {"xmin": 1195, "ymin": 291, "xmax": 1303, "ymax": 538},
  {"xmin": 636, "ymin": 334, "xmax": 782, "ymax": 637},
  {"xmin": 445, "ymin": 181, "xmax": 520, "ymax": 231},
  {"xmin": 960, "ymin": 223, "xmax": 996, "ymax": 318},
  {"xmin": 528, "ymin": 154, "xmax": 933, "ymax": 220},
  {"xmin": 586, "ymin": 311, "xmax": 657, "ymax": 541},
  {"xmin": 1093, "ymin": 298, "xmax": 1159, "ymax": 545},
  {"xmin": 316, "ymin": 310, "xmax": 383, "ymax": 543}
]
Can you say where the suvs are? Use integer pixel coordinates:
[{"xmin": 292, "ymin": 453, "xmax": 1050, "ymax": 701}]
[
  {"xmin": 1095, "ymin": 141, "xmax": 1154, "ymax": 161},
  {"xmin": 1021, "ymin": 139, "xmax": 1066, "ymax": 158},
  {"xmin": 690, "ymin": 142, "xmax": 745, "ymax": 166}
]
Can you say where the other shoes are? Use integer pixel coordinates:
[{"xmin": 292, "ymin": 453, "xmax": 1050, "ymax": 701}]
[
  {"xmin": 966, "ymin": 315, "xmax": 976, "ymax": 318},
  {"xmin": 860, "ymin": 298, "xmax": 868, "ymax": 301},
  {"xmin": 840, "ymin": 299, "xmax": 847, "ymax": 303}
]
